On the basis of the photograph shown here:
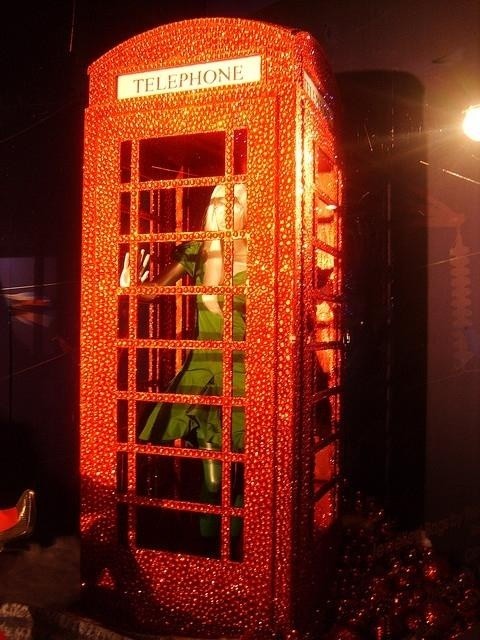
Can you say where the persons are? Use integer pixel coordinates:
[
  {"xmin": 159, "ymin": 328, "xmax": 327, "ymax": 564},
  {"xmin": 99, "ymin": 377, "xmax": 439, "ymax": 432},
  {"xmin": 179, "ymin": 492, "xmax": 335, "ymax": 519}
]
[{"xmin": 120, "ymin": 182, "xmax": 248, "ymax": 560}]
